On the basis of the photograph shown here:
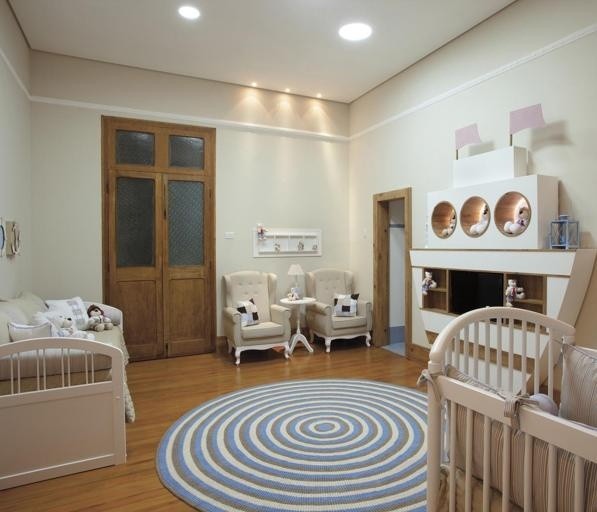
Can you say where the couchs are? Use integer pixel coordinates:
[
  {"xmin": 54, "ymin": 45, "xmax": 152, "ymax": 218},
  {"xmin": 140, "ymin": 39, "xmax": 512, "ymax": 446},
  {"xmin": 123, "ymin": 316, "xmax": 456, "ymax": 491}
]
[{"xmin": 0, "ymin": 292, "xmax": 136, "ymax": 425}]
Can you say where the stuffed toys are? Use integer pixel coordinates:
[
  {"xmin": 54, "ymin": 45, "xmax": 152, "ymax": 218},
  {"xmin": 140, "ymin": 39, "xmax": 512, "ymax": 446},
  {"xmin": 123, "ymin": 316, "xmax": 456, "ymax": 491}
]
[
  {"xmin": 504, "ymin": 197, "xmax": 530, "ymax": 234},
  {"xmin": 33, "ymin": 311, "xmax": 95, "ymax": 349},
  {"xmin": 442, "ymin": 210, "xmax": 456, "ymax": 237},
  {"xmin": 422, "ymin": 271, "xmax": 438, "ymax": 295},
  {"xmin": 470, "ymin": 204, "xmax": 490, "ymax": 235},
  {"xmin": 88, "ymin": 305, "xmax": 113, "ymax": 331},
  {"xmin": 506, "ymin": 278, "xmax": 526, "ymax": 307}
]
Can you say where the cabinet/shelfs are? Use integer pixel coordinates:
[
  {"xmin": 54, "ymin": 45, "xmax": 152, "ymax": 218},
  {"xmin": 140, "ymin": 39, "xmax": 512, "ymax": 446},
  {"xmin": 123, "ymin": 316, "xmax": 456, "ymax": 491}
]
[{"xmin": 409, "ymin": 175, "xmax": 597, "ymax": 334}]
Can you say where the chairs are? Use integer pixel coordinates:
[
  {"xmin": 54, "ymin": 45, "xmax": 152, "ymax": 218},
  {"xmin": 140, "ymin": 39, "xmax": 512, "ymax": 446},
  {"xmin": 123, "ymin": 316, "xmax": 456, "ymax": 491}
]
[
  {"xmin": 222, "ymin": 270, "xmax": 292, "ymax": 364},
  {"xmin": 306, "ymin": 266, "xmax": 373, "ymax": 353}
]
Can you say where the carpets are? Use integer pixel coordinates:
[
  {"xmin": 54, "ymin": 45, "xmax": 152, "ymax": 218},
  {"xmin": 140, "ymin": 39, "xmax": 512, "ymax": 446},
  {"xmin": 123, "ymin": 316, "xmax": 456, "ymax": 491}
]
[{"xmin": 157, "ymin": 377, "xmax": 449, "ymax": 512}]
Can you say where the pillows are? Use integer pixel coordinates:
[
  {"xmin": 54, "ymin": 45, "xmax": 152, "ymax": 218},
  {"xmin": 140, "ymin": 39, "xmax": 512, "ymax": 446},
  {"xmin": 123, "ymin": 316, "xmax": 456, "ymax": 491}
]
[
  {"xmin": 552, "ymin": 333, "xmax": 597, "ymax": 426},
  {"xmin": 8, "ymin": 297, "xmax": 88, "ymax": 350},
  {"xmin": 331, "ymin": 292, "xmax": 359, "ymax": 317},
  {"xmin": 236, "ymin": 298, "xmax": 259, "ymax": 327}
]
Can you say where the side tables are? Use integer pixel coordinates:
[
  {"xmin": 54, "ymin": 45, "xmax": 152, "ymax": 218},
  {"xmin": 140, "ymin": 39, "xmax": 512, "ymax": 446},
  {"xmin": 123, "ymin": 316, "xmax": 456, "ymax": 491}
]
[{"xmin": 279, "ymin": 296, "xmax": 316, "ymax": 354}]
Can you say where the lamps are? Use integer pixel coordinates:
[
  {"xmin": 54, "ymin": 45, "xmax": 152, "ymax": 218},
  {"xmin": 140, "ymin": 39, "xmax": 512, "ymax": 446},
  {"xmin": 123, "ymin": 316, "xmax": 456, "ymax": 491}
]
[{"xmin": 287, "ymin": 264, "xmax": 304, "ymax": 298}]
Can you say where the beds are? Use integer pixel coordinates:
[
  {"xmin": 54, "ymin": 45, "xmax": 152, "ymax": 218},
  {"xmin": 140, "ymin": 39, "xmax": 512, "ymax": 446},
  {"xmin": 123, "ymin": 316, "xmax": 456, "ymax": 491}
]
[{"xmin": 423, "ymin": 305, "xmax": 596, "ymax": 512}]
